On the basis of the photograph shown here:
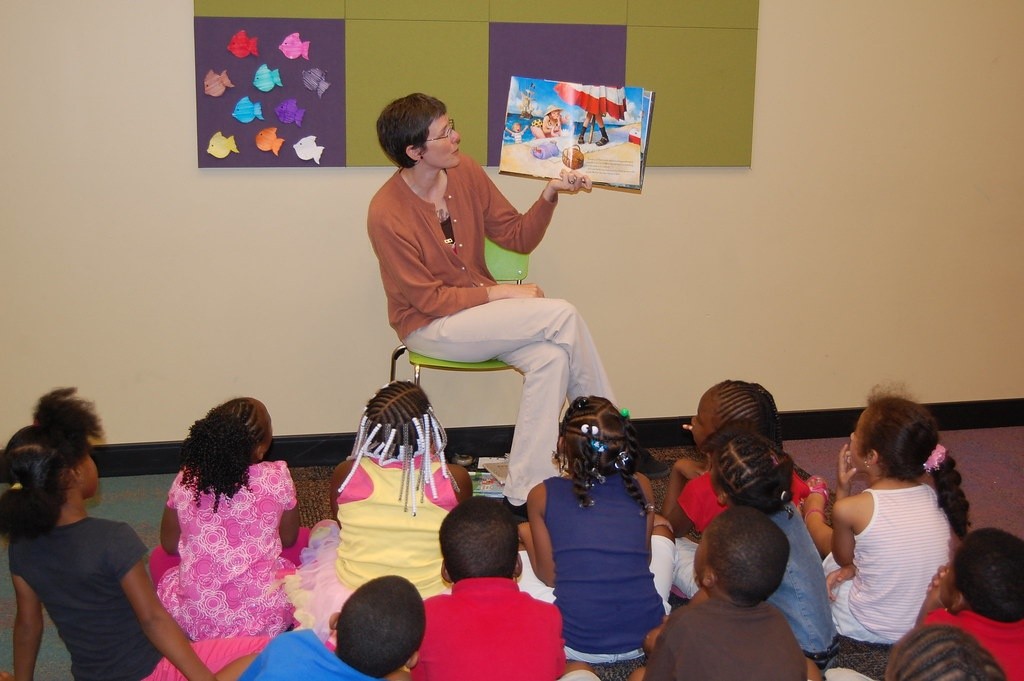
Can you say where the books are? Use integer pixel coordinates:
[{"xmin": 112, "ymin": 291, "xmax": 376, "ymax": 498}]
[{"xmin": 498, "ymin": 76, "xmax": 654, "ymax": 190}]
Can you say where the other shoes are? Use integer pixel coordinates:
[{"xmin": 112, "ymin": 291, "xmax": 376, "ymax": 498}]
[
  {"xmin": 502, "ymin": 496, "xmax": 530, "ymax": 524},
  {"xmin": 626, "ymin": 437, "xmax": 671, "ymax": 479}
]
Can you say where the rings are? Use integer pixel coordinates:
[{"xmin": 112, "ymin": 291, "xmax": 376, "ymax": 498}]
[{"xmin": 646, "ymin": 635, "xmax": 649, "ymax": 638}]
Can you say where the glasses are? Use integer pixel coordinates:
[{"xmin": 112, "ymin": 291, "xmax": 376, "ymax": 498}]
[{"xmin": 426, "ymin": 117, "xmax": 456, "ymax": 141}]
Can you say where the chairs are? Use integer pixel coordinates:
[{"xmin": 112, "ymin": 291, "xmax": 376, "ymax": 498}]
[{"xmin": 390, "ymin": 236, "xmax": 529, "ymax": 387}]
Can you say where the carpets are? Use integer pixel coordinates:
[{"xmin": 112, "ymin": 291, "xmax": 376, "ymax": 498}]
[{"xmin": 284, "ymin": 446, "xmax": 887, "ymax": 681}]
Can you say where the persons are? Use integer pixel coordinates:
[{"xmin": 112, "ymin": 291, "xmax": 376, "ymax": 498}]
[
  {"xmin": 367, "ymin": 93, "xmax": 615, "ymax": 520},
  {"xmin": 299, "ymin": 379, "xmax": 473, "ymax": 612},
  {"xmin": 802, "ymin": 385, "xmax": 970, "ymax": 644},
  {"xmin": 239, "ymin": 575, "xmax": 427, "ymax": 681},
  {"xmin": 149, "ymin": 397, "xmax": 310, "ymax": 642},
  {"xmin": 517, "ymin": 396, "xmax": 675, "ymax": 663},
  {"xmin": 630, "ymin": 506, "xmax": 808, "ymax": 681},
  {"xmin": 408, "ymin": 496, "xmax": 602, "ymax": 681},
  {"xmin": 915, "ymin": 527, "xmax": 1024, "ymax": 681},
  {"xmin": 662, "ymin": 380, "xmax": 811, "ymax": 599},
  {"xmin": 644, "ymin": 433, "xmax": 841, "ymax": 675},
  {"xmin": 884, "ymin": 623, "xmax": 1007, "ymax": 681},
  {"xmin": 0, "ymin": 388, "xmax": 271, "ymax": 681}
]
